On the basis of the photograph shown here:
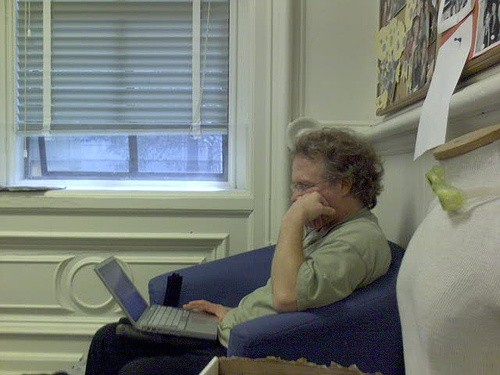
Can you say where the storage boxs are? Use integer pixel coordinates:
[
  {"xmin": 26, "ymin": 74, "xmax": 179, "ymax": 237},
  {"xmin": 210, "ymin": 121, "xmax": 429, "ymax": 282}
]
[{"xmin": 200, "ymin": 356, "xmax": 383, "ymax": 375}]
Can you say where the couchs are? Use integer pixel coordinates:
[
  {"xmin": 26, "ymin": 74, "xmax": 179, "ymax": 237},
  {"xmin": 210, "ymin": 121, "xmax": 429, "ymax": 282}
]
[{"xmin": 148, "ymin": 241, "xmax": 406, "ymax": 375}]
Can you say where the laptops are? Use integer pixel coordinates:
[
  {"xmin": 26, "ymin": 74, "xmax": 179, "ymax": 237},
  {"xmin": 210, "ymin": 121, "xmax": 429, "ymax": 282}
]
[{"xmin": 94, "ymin": 256, "xmax": 219, "ymax": 340}]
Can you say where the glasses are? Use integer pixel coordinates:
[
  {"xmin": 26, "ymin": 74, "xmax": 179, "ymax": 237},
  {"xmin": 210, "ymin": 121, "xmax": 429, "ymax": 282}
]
[{"xmin": 290, "ymin": 175, "xmax": 341, "ymax": 193}]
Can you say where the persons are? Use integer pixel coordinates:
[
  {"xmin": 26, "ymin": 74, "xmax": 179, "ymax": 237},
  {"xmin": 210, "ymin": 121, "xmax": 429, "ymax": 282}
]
[
  {"xmin": 86, "ymin": 126, "xmax": 392, "ymax": 375},
  {"xmin": 408, "ymin": 0, "xmax": 500, "ymax": 94}
]
